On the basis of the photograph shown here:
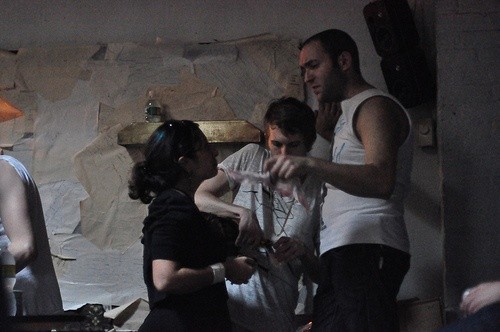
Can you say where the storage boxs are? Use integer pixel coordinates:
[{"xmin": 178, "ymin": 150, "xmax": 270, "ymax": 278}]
[{"xmin": 395, "ymin": 298, "xmax": 444, "ymax": 332}]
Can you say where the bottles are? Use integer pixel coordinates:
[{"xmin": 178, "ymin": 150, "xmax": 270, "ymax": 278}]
[
  {"xmin": 0, "ymin": 242, "xmax": 18, "ymax": 316},
  {"xmin": 143, "ymin": 90, "xmax": 161, "ymax": 122}
]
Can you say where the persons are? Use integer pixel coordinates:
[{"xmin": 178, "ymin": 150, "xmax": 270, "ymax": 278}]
[
  {"xmin": 128, "ymin": 118, "xmax": 257, "ymax": 332},
  {"xmin": 459, "ymin": 281, "xmax": 500, "ymax": 317},
  {"xmin": 194, "ymin": 97, "xmax": 323, "ymax": 332},
  {"xmin": 263, "ymin": 29, "xmax": 413, "ymax": 332},
  {"xmin": 0, "ymin": 156, "xmax": 63, "ymax": 316}
]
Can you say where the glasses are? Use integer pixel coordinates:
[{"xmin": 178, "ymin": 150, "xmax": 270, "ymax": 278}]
[{"xmin": 168, "ymin": 119, "xmax": 187, "ymax": 149}]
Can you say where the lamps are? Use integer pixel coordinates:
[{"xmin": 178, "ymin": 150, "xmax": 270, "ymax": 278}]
[{"xmin": 0, "ymin": 98, "xmax": 23, "ymax": 123}]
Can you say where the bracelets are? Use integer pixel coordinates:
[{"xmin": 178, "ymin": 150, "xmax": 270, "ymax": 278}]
[{"xmin": 210, "ymin": 263, "xmax": 225, "ymax": 284}]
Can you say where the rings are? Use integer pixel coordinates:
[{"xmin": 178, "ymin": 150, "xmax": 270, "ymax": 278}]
[{"xmin": 464, "ymin": 290, "xmax": 469, "ymax": 296}]
